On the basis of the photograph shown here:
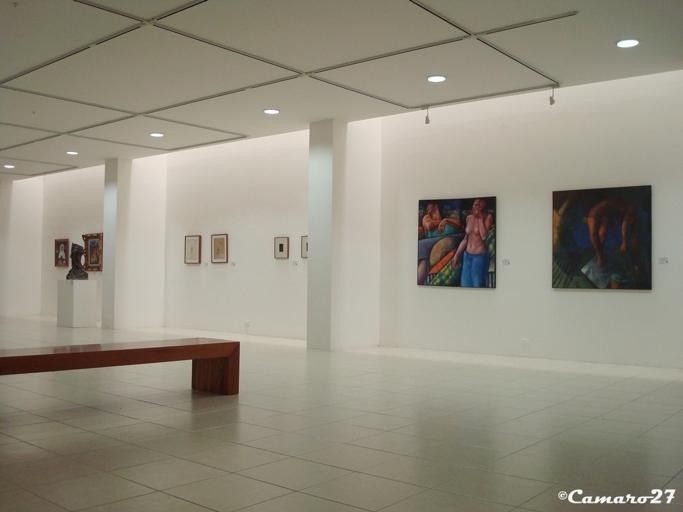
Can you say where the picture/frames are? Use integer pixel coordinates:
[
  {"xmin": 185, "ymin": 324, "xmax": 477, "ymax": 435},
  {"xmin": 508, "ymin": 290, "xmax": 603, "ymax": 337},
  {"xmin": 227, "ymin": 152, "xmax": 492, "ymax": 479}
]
[
  {"xmin": 273, "ymin": 235, "xmax": 311, "ymax": 261},
  {"xmin": 184, "ymin": 233, "xmax": 228, "ymax": 266},
  {"xmin": 52, "ymin": 233, "xmax": 102, "ymax": 273}
]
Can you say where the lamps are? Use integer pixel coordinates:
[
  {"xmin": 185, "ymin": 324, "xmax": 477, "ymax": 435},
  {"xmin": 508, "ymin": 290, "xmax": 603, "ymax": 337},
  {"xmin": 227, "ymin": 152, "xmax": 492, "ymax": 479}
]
[
  {"xmin": 423, "ymin": 106, "xmax": 432, "ymax": 124},
  {"xmin": 549, "ymin": 89, "xmax": 554, "ymax": 107}
]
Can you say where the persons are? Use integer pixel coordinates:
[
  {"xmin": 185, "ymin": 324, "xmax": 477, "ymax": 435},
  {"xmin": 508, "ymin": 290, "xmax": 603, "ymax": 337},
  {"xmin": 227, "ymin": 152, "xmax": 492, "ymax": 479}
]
[
  {"xmin": 417, "ymin": 199, "xmax": 465, "ymax": 286},
  {"xmin": 580, "ymin": 195, "xmax": 643, "ymax": 268},
  {"xmin": 450, "ymin": 197, "xmax": 494, "ymax": 288}
]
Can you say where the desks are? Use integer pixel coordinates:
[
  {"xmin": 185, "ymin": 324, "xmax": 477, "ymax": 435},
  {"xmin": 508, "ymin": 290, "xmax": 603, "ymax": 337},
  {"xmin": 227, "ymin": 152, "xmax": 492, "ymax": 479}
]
[{"xmin": 1, "ymin": 337, "xmax": 241, "ymax": 398}]
